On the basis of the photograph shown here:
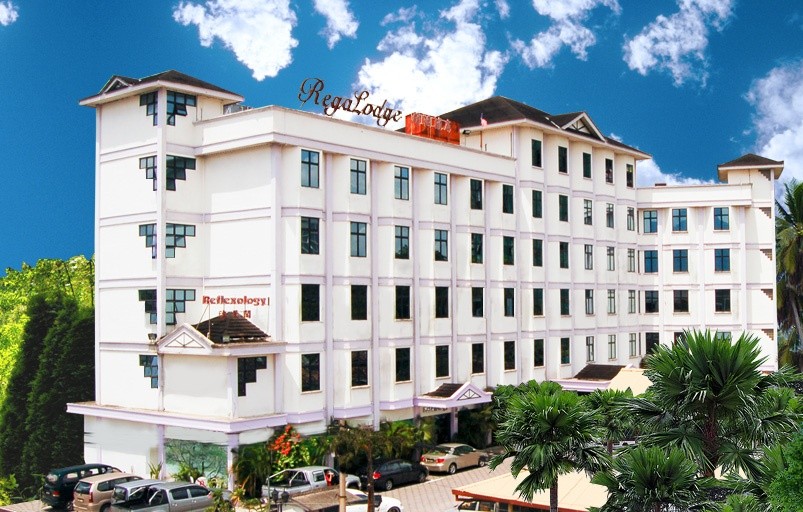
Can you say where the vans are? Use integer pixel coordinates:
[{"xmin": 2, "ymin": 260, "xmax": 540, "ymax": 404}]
[{"xmin": 39, "ymin": 463, "xmax": 123, "ymax": 512}]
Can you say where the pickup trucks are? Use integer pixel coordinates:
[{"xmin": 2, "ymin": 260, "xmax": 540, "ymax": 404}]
[
  {"xmin": 113, "ymin": 481, "xmax": 236, "ymax": 512},
  {"xmin": 260, "ymin": 465, "xmax": 361, "ymax": 506}
]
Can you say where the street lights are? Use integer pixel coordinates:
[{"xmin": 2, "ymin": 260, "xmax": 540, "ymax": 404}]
[{"xmin": 272, "ymin": 486, "xmax": 289, "ymax": 512}]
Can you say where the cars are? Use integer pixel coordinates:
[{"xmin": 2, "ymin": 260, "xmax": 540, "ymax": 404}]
[
  {"xmin": 279, "ymin": 486, "xmax": 402, "ymax": 512},
  {"xmin": 419, "ymin": 442, "xmax": 490, "ymax": 476},
  {"xmin": 353, "ymin": 457, "xmax": 428, "ymax": 491},
  {"xmin": 71, "ymin": 471, "xmax": 147, "ymax": 512},
  {"xmin": 110, "ymin": 479, "xmax": 166, "ymax": 506}
]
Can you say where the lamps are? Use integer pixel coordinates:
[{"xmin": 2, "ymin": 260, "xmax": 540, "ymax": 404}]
[
  {"xmin": 148, "ymin": 333, "xmax": 157, "ymax": 342},
  {"xmin": 223, "ymin": 335, "xmax": 230, "ymax": 344},
  {"xmin": 462, "ymin": 129, "xmax": 471, "ymax": 135}
]
